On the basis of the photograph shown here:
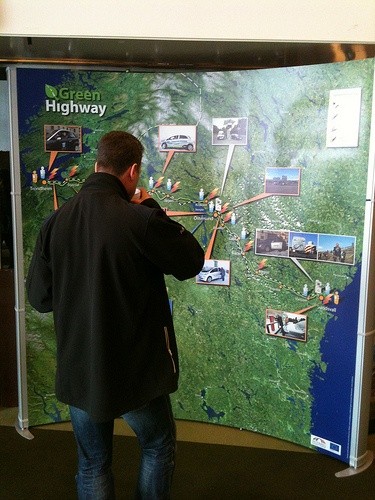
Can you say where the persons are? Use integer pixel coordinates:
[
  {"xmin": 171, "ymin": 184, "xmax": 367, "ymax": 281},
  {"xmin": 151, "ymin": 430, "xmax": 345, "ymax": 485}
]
[
  {"xmin": 275, "ymin": 315, "xmax": 285, "ymax": 336},
  {"xmin": 25, "ymin": 131, "xmax": 205, "ymax": 500},
  {"xmin": 220, "ymin": 267, "xmax": 225, "ymax": 281},
  {"xmin": 333, "ymin": 243, "xmax": 345, "ymax": 263}
]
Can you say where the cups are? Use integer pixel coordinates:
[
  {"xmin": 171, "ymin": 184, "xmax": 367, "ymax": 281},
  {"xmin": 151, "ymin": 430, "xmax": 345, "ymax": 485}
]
[{"xmin": 132, "ymin": 189, "xmax": 140, "ymax": 205}]
